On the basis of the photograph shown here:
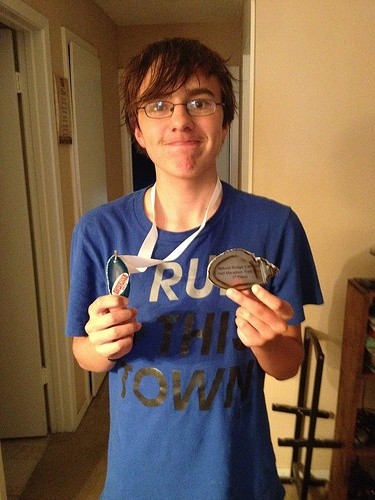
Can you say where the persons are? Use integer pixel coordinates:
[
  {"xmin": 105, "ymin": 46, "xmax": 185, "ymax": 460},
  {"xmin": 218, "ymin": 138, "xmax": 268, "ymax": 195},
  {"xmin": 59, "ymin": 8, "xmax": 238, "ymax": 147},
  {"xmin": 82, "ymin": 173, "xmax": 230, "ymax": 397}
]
[{"xmin": 65, "ymin": 36, "xmax": 324, "ymax": 500}]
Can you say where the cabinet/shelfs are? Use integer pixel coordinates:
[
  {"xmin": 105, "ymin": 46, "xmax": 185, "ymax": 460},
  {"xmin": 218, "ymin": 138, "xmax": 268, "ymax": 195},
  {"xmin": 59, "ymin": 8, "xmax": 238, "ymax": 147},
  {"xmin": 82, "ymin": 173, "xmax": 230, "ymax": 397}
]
[{"xmin": 325, "ymin": 277, "xmax": 375, "ymax": 500}]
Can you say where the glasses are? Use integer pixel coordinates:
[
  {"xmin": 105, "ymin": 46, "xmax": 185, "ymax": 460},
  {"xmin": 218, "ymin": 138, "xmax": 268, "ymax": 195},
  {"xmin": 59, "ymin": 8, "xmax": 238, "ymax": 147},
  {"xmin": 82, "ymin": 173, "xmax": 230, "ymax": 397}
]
[{"xmin": 135, "ymin": 98, "xmax": 224, "ymax": 119}]
